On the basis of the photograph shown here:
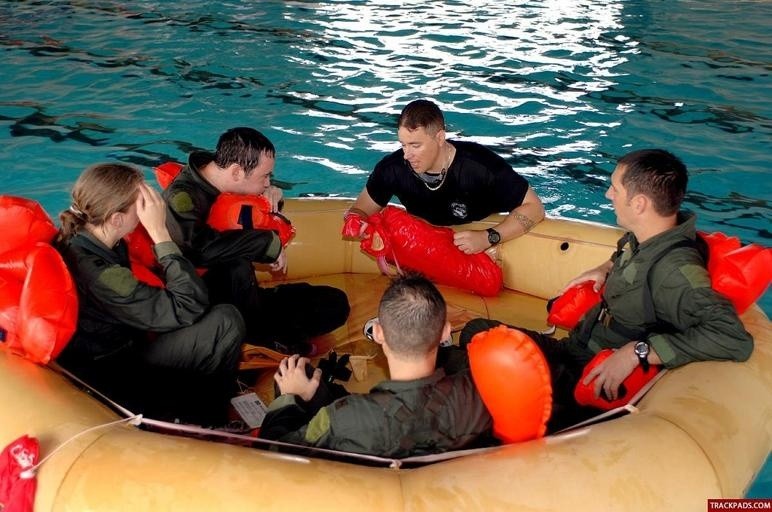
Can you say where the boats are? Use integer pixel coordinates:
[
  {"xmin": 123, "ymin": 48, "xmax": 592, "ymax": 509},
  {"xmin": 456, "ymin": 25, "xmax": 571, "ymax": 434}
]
[{"xmin": 0, "ymin": 194, "xmax": 772, "ymax": 511}]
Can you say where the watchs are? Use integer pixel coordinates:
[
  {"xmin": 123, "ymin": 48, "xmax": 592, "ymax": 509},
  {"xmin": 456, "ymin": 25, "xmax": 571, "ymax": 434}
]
[
  {"xmin": 633, "ymin": 338, "xmax": 651, "ymax": 373},
  {"xmin": 484, "ymin": 227, "xmax": 501, "ymax": 247}
]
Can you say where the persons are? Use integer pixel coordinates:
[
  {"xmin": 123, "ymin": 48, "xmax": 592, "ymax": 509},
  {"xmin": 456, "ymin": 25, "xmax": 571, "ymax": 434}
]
[
  {"xmin": 342, "ymin": 98, "xmax": 555, "ymax": 257},
  {"xmin": 158, "ymin": 125, "xmax": 353, "ymax": 358},
  {"xmin": 40, "ymin": 160, "xmax": 255, "ymax": 439},
  {"xmin": 252, "ymin": 271, "xmax": 501, "ymax": 472},
  {"xmin": 458, "ymin": 149, "xmax": 753, "ymax": 435}
]
